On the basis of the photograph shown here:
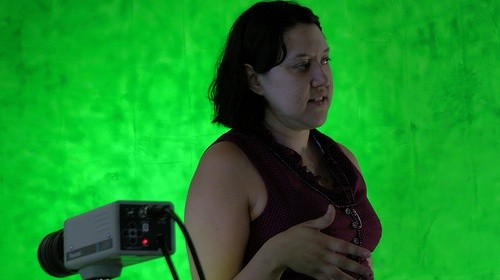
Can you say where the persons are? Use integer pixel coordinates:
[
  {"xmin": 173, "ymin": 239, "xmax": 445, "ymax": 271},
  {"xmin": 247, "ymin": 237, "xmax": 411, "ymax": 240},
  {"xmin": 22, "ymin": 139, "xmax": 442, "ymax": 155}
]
[{"xmin": 182, "ymin": 0, "xmax": 384, "ymax": 280}]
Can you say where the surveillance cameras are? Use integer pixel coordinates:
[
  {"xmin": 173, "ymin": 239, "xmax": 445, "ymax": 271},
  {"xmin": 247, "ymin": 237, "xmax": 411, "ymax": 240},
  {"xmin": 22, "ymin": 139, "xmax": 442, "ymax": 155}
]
[{"xmin": 38, "ymin": 200, "xmax": 175, "ymax": 279}]
